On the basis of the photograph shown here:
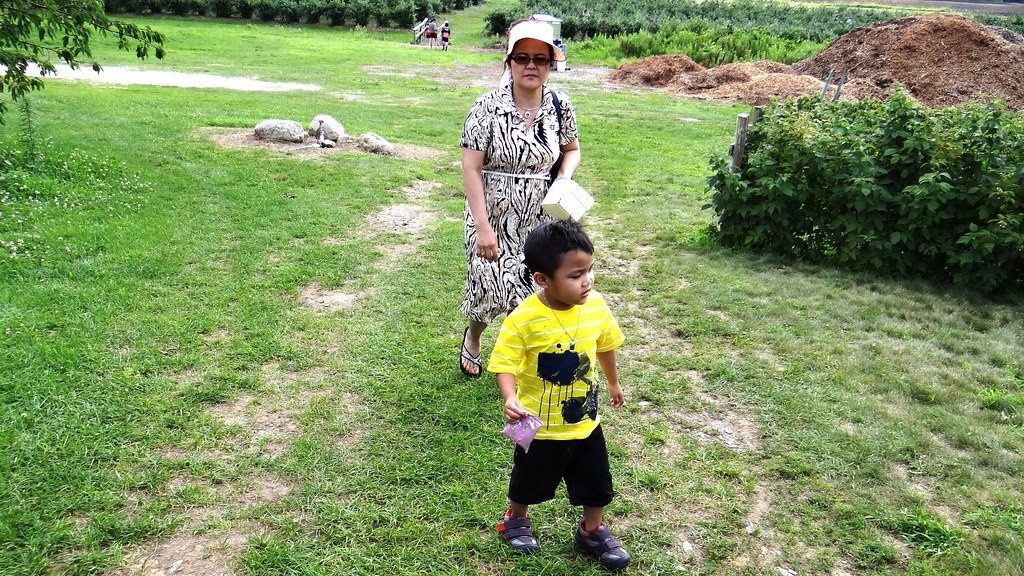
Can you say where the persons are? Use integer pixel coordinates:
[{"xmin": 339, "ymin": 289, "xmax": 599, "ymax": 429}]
[
  {"xmin": 458, "ymin": 19, "xmax": 582, "ymax": 379},
  {"xmin": 439, "ymin": 22, "xmax": 451, "ymax": 51},
  {"xmin": 426, "ymin": 18, "xmax": 439, "ymax": 48},
  {"xmin": 487, "ymin": 217, "xmax": 632, "ymax": 569},
  {"xmin": 424, "ymin": 24, "xmax": 430, "ymax": 45}
]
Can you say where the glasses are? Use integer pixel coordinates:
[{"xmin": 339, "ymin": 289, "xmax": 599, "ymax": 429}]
[{"xmin": 511, "ymin": 53, "xmax": 553, "ymax": 66}]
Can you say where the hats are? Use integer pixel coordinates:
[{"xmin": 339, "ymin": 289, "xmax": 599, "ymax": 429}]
[{"xmin": 506, "ymin": 19, "xmax": 567, "ymax": 62}]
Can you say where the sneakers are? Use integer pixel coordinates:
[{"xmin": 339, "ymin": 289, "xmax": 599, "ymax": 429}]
[
  {"xmin": 575, "ymin": 517, "xmax": 631, "ymax": 571},
  {"xmin": 497, "ymin": 507, "xmax": 539, "ymax": 553}
]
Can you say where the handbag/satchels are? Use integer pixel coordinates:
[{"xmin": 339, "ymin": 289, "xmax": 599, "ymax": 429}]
[{"xmin": 550, "ymin": 88, "xmax": 565, "ymax": 178}]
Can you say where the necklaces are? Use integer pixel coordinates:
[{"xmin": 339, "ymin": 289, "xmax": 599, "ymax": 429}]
[
  {"xmin": 516, "ymin": 100, "xmax": 546, "ymax": 118},
  {"xmin": 542, "ymin": 291, "xmax": 580, "ymax": 344}
]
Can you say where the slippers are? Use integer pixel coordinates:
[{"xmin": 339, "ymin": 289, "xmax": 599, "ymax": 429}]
[{"xmin": 460, "ymin": 326, "xmax": 483, "ymax": 377}]
